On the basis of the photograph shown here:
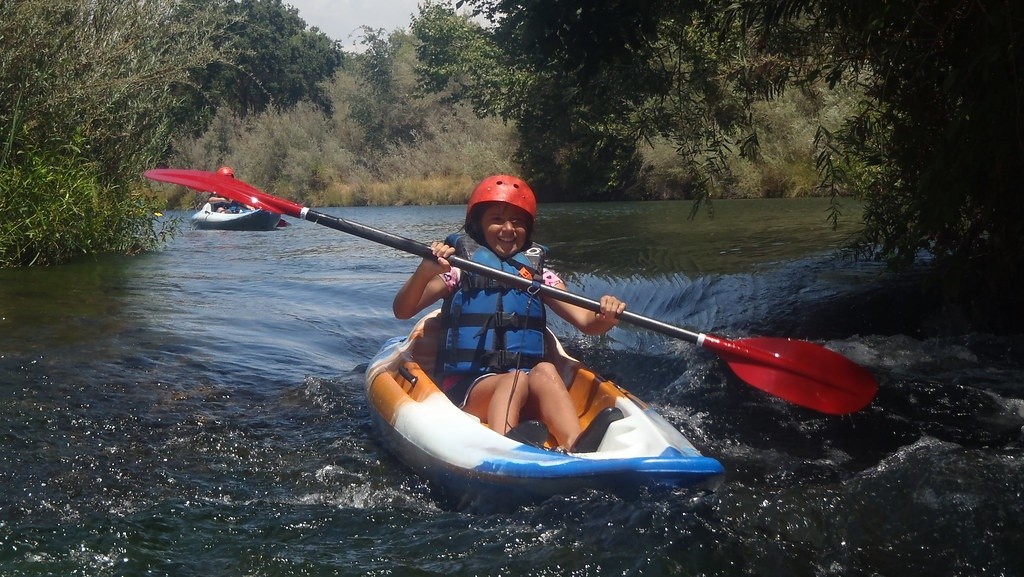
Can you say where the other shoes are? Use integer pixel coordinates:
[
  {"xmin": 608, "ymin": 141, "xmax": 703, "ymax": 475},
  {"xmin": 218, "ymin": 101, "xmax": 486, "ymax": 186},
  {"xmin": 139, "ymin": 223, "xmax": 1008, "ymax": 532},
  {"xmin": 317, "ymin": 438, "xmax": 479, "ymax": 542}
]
[
  {"xmin": 503, "ymin": 420, "xmax": 549, "ymax": 448},
  {"xmin": 569, "ymin": 406, "xmax": 625, "ymax": 452}
]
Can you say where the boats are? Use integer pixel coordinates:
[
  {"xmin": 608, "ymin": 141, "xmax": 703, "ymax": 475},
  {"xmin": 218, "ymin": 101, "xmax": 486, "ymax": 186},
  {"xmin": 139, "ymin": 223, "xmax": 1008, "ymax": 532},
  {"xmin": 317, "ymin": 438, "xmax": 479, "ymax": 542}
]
[
  {"xmin": 191, "ymin": 202, "xmax": 281, "ymax": 230},
  {"xmin": 366, "ymin": 307, "xmax": 726, "ymax": 498}
]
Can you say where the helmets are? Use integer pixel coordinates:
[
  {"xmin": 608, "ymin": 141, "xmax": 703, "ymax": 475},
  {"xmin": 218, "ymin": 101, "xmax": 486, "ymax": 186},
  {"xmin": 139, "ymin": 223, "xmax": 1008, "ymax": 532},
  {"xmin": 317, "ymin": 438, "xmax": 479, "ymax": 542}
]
[
  {"xmin": 215, "ymin": 166, "xmax": 234, "ymax": 179},
  {"xmin": 467, "ymin": 175, "xmax": 537, "ymax": 224}
]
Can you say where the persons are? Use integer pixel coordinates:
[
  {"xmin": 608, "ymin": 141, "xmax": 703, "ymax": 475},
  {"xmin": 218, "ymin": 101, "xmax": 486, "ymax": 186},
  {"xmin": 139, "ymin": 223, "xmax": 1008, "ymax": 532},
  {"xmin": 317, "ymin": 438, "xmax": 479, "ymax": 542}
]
[
  {"xmin": 393, "ymin": 174, "xmax": 626, "ymax": 453},
  {"xmin": 208, "ymin": 165, "xmax": 255, "ymax": 214}
]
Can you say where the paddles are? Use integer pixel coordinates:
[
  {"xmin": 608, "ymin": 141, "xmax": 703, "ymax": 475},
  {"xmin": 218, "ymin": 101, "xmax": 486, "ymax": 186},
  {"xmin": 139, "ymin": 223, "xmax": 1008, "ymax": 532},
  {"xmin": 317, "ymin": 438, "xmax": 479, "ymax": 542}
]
[{"xmin": 145, "ymin": 168, "xmax": 882, "ymax": 417}]
